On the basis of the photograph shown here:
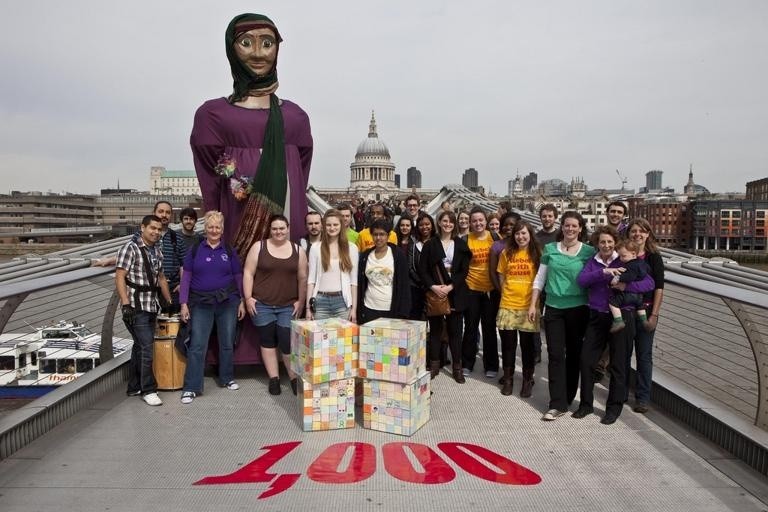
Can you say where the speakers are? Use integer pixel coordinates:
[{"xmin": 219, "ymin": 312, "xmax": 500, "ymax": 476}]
[
  {"xmin": 427, "ymin": 360, "xmax": 440, "ymax": 379},
  {"xmin": 501, "ymin": 366, "xmax": 514, "ymax": 395},
  {"xmin": 520, "ymin": 368, "xmax": 535, "ymax": 397},
  {"xmin": 453, "ymin": 361, "xmax": 465, "ymax": 384}
]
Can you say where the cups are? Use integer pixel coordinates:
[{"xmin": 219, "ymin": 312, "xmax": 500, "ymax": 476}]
[
  {"xmin": 309, "ymin": 296, "xmax": 317, "ymax": 313},
  {"xmin": 408, "ymin": 204, "xmax": 418, "ymax": 208}
]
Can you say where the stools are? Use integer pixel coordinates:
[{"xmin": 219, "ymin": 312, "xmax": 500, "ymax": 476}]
[{"xmin": 318, "ymin": 291, "xmax": 342, "ymax": 296}]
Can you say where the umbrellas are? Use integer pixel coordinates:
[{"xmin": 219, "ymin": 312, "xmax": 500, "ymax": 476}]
[
  {"xmin": 601, "ymin": 412, "xmax": 619, "ymax": 424},
  {"xmin": 634, "ymin": 399, "xmax": 648, "ymax": 412},
  {"xmin": 572, "ymin": 407, "xmax": 593, "ymax": 418}
]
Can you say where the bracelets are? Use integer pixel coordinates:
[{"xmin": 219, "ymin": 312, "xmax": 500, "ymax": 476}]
[
  {"xmin": 306, "ymin": 308, "xmax": 310, "ymax": 309},
  {"xmin": 246, "ymin": 296, "xmax": 252, "ymax": 301},
  {"xmin": 652, "ymin": 314, "xmax": 657, "ymax": 317},
  {"xmin": 240, "ymin": 298, "xmax": 246, "ymax": 304}
]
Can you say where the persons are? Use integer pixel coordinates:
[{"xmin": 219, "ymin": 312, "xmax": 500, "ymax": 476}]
[
  {"xmin": 349, "ymin": 198, "xmax": 406, "ymax": 233},
  {"xmin": 190, "ymin": 12, "xmax": 313, "ymax": 367},
  {"xmin": 355, "ymin": 204, "xmax": 470, "ymax": 383},
  {"xmin": 132, "ymin": 201, "xmax": 186, "ymax": 314},
  {"xmin": 243, "ymin": 215, "xmax": 308, "ymax": 395},
  {"xmin": 571, "ymin": 202, "xmax": 663, "ymax": 424},
  {"xmin": 179, "ymin": 210, "xmax": 247, "ymax": 404},
  {"xmin": 117, "ymin": 215, "xmax": 177, "ymax": 406},
  {"xmin": 435, "ymin": 200, "xmax": 471, "ymax": 233},
  {"xmin": 393, "ymin": 197, "xmax": 435, "ymax": 237},
  {"xmin": 294, "ymin": 212, "xmax": 322, "ymax": 260},
  {"xmin": 461, "ymin": 204, "xmax": 596, "ymax": 420},
  {"xmin": 498, "ymin": 202, "xmax": 512, "ymax": 215},
  {"xmin": 306, "ymin": 208, "xmax": 359, "ymax": 324},
  {"xmin": 179, "ymin": 207, "xmax": 198, "ymax": 247}
]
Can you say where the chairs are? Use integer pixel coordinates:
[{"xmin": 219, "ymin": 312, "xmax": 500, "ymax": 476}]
[
  {"xmin": 128, "ymin": 389, "xmax": 143, "ymax": 396},
  {"xmin": 484, "ymin": 371, "xmax": 497, "ymax": 378},
  {"xmin": 143, "ymin": 392, "xmax": 163, "ymax": 406},
  {"xmin": 220, "ymin": 380, "xmax": 239, "ymax": 390},
  {"xmin": 269, "ymin": 377, "xmax": 281, "ymax": 395},
  {"xmin": 181, "ymin": 390, "xmax": 196, "ymax": 404},
  {"xmin": 543, "ymin": 409, "xmax": 565, "ymax": 419},
  {"xmin": 461, "ymin": 368, "xmax": 471, "ymax": 376},
  {"xmin": 291, "ymin": 378, "xmax": 298, "ymax": 395}
]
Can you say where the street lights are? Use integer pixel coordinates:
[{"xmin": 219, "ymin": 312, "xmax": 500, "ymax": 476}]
[{"xmin": 426, "ymin": 289, "xmax": 451, "ymax": 317}]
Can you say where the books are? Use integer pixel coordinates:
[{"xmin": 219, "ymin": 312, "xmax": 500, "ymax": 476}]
[
  {"xmin": 296, "ymin": 373, "xmax": 356, "ymax": 432},
  {"xmin": 359, "ymin": 317, "xmax": 427, "ymax": 384},
  {"xmin": 363, "ymin": 371, "xmax": 431, "ymax": 438},
  {"xmin": 287, "ymin": 317, "xmax": 359, "ymax": 385}
]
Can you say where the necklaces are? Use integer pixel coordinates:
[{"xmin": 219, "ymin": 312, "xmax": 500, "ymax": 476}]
[{"xmin": 563, "ymin": 241, "xmax": 578, "ymax": 251}]
